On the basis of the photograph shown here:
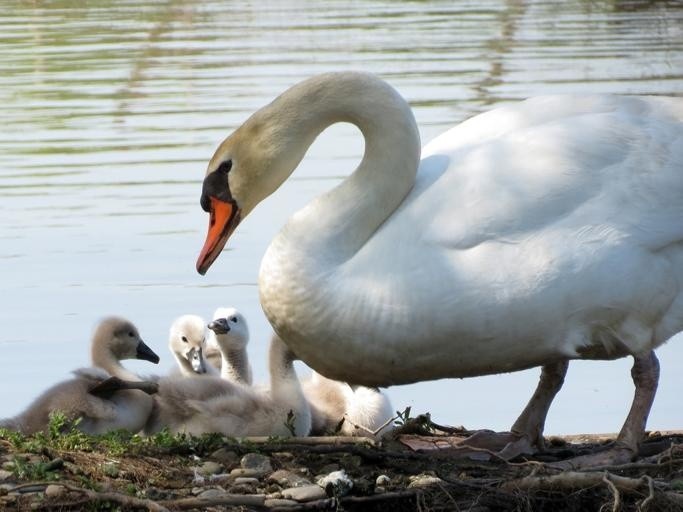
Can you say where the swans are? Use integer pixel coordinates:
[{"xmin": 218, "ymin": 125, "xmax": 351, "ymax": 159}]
[
  {"xmin": 195, "ymin": 68, "xmax": 683, "ymax": 475},
  {"xmin": 147, "ymin": 310, "xmax": 392, "ymax": 439},
  {"xmin": 1, "ymin": 315, "xmax": 159, "ymax": 436}
]
